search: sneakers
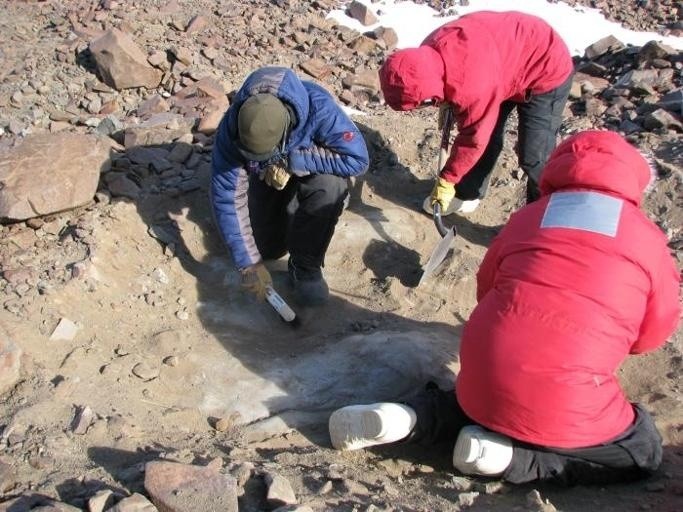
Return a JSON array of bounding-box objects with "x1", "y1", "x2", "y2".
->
[
  {"x1": 429, "y1": 176, "x2": 456, "y2": 213},
  {"x1": 423, "y1": 196, "x2": 481, "y2": 216},
  {"x1": 452, "y1": 425, "x2": 513, "y2": 477},
  {"x1": 329, "y1": 402, "x2": 417, "y2": 451},
  {"x1": 288, "y1": 256, "x2": 329, "y2": 305}
]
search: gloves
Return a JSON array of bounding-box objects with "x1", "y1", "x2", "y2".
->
[
  {"x1": 240, "y1": 263, "x2": 273, "y2": 304},
  {"x1": 260, "y1": 154, "x2": 294, "y2": 190}
]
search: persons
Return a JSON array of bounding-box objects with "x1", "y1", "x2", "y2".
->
[
  {"x1": 329, "y1": 132, "x2": 681, "y2": 487},
  {"x1": 378, "y1": 11, "x2": 573, "y2": 216},
  {"x1": 210, "y1": 67, "x2": 369, "y2": 307}
]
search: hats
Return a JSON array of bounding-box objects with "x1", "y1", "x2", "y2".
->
[{"x1": 231, "y1": 93, "x2": 291, "y2": 161}]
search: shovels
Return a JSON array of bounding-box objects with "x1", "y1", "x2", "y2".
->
[{"x1": 418, "y1": 108, "x2": 457, "y2": 288}]
